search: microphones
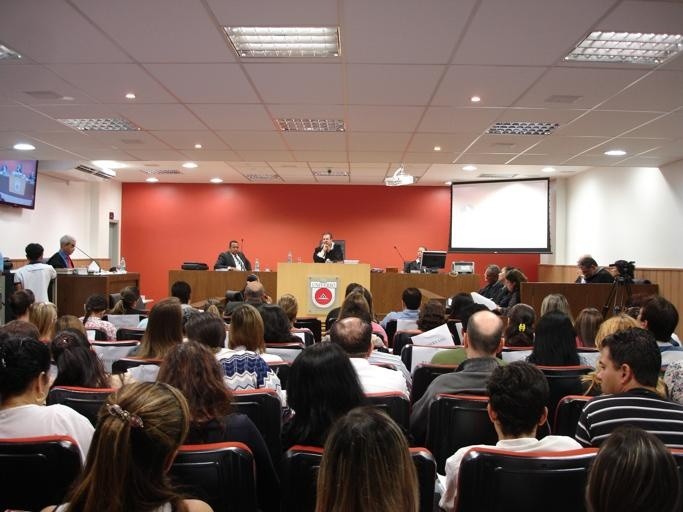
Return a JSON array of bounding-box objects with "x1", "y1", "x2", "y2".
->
[
  {"x1": 394, "y1": 246, "x2": 407, "y2": 273},
  {"x1": 241, "y1": 238, "x2": 244, "y2": 272},
  {"x1": 71, "y1": 244, "x2": 101, "y2": 275}
]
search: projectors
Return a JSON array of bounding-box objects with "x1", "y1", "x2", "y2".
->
[{"x1": 386, "y1": 175, "x2": 413, "y2": 187}]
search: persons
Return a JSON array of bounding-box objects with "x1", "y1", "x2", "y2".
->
[
  {"x1": 477, "y1": 265, "x2": 527, "y2": 313},
  {"x1": 1, "y1": 161, "x2": 35, "y2": 181},
  {"x1": 402, "y1": 246, "x2": 437, "y2": 273},
  {"x1": 313, "y1": 233, "x2": 343, "y2": 263},
  {"x1": 13, "y1": 243, "x2": 55, "y2": 302},
  {"x1": 214, "y1": 240, "x2": 252, "y2": 271},
  {"x1": 0, "y1": 274, "x2": 683, "y2": 512},
  {"x1": 47, "y1": 236, "x2": 76, "y2": 268},
  {"x1": 575, "y1": 255, "x2": 635, "y2": 283}
]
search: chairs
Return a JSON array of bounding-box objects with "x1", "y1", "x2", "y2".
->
[{"x1": 0, "y1": 308, "x2": 683, "y2": 512}]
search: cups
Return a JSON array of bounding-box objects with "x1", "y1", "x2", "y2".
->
[
  {"x1": 263, "y1": 265, "x2": 273, "y2": 272},
  {"x1": 297, "y1": 256, "x2": 301, "y2": 263},
  {"x1": 76, "y1": 268, "x2": 88, "y2": 275}
]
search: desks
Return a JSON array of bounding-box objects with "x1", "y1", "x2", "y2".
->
[
  {"x1": 168, "y1": 269, "x2": 277, "y2": 306},
  {"x1": 370, "y1": 271, "x2": 480, "y2": 317},
  {"x1": 58, "y1": 272, "x2": 140, "y2": 318},
  {"x1": 520, "y1": 282, "x2": 659, "y2": 320}
]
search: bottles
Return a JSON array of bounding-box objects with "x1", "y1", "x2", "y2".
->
[
  {"x1": 286, "y1": 248, "x2": 293, "y2": 262},
  {"x1": 119, "y1": 255, "x2": 125, "y2": 273},
  {"x1": 253, "y1": 255, "x2": 259, "y2": 272}
]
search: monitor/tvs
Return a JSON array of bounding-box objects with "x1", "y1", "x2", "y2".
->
[
  {"x1": 420, "y1": 251, "x2": 447, "y2": 273},
  {"x1": 0, "y1": 160, "x2": 38, "y2": 209}
]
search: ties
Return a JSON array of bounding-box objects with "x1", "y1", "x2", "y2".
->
[
  {"x1": 235, "y1": 255, "x2": 245, "y2": 271},
  {"x1": 66, "y1": 256, "x2": 73, "y2": 268}
]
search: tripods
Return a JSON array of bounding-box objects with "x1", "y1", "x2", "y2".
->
[{"x1": 602, "y1": 276, "x2": 632, "y2": 320}]
